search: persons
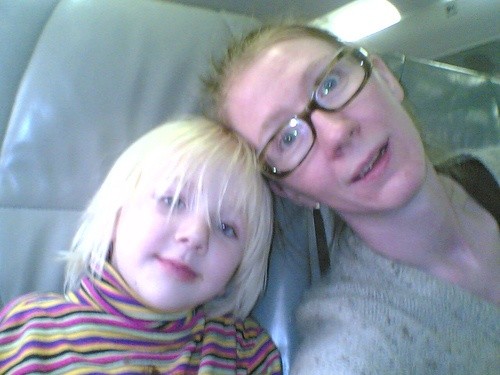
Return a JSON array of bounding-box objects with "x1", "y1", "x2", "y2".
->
[
  {"x1": 0, "y1": 115, "x2": 284, "y2": 375},
  {"x1": 196, "y1": 22, "x2": 500, "y2": 375}
]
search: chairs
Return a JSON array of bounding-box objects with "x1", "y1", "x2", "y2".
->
[{"x1": 1, "y1": 0, "x2": 500, "y2": 375}]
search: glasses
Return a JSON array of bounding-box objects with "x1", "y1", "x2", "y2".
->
[{"x1": 255, "y1": 44, "x2": 374, "y2": 183}]
